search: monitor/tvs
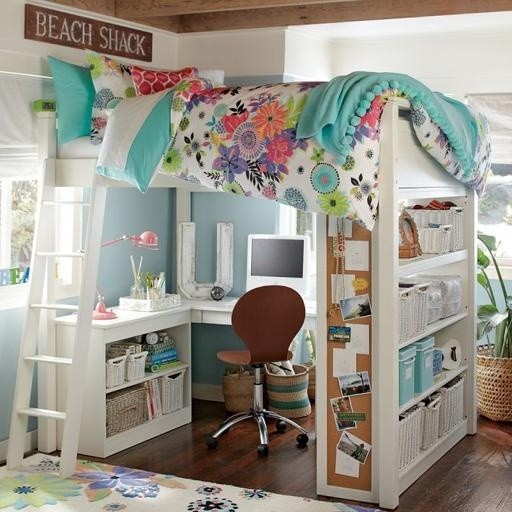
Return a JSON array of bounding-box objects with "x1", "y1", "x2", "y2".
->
[{"x1": 246, "y1": 233, "x2": 311, "y2": 299}]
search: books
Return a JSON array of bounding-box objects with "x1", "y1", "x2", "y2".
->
[
  {"x1": 107, "y1": 343, "x2": 141, "y2": 363},
  {"x1": 141, "y1": 379, "x2": 164, "y2": 421}
]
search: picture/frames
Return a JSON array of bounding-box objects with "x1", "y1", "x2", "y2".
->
[{"x1": 399, "y1": 211, "x2": 417, "y2": 245}]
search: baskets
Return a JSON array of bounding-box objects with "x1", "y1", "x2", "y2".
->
[
  {"x1": 105, "y1": 386, "x2": 145, "y2": 438},
  {"x1": 223, "y1": 370, "x2": 264, "y2": 412},
  {"x1": 422, "y1": 391, "x2": 444, "y2": 450},
  {"x1": 417, "y1": 222, "x2": 453, "y2": 255},
  {"x1": 105, "y1": 353, "x2": 127, "y2": 388},
  {"x1": 397, "y1": 404, "x2": 422, "y2": 474},
  {"x1": 405, "y1": 206, "x2": 464, "y2": 253},
  {"x1": 415, "y1": 281, "x2": 431, "y2": 335},
  {"x1": 124, "y1": 351, "x2": 149, "y2": 382},
  {"x1": 475, "y1": 341, "x2": 512, "y2": 424},
  {"x1": 160, "y1": 369, "x2": 186, "y2": 416},
  {"x1": 439, "y1": 374, "x2": 464, "y2": 438},
  {"x1": 399, "y1": 287, "x2": 416, "y2": 344},
  {"x1": 305, "y1": 363, "x2": 315, "y2": 399},
  {"x1": 264, "y1": 362, "x2": 315, "y2": 419}
]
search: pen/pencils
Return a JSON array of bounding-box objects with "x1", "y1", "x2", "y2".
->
[{"x1": 129, "y1": 254, "x2": 143, "y2": 300}]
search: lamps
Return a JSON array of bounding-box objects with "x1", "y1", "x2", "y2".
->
[{"x1": 80, "y1": 231, "x2": 159, "y2": 320}]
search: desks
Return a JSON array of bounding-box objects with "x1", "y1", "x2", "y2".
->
[{"x1": 192, "y1": 296, "x2": 316, "y2": 330}]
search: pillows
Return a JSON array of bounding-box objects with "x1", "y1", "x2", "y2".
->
[
  {"x1": 127, "y1": 65, "x2": 199, "y2": 97},
  {"x1": 46, "y1": 56, "x2": 96, "y2": 145},
  {"x1": 85, "y1": 48, "x2": 200, "y2": 147}
]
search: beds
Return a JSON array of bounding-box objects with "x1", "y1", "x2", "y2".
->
[{"x1": 34, "y1": 48, "x2": 493, "y2": 232}]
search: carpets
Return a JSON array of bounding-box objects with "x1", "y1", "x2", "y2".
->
[{"x1": 0, "y1": 453, "x2": 387, "y2": 512}]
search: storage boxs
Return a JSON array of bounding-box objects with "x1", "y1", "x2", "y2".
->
[
  {"x1": 399, "y1": 346, "x2": 416, "y2": 406},
  {"x1": 399, "y1": 246, "x2": 418, "y2": 258},
  {"x1": 409, "y1": 336, "x2": 434, "y2": 393}
]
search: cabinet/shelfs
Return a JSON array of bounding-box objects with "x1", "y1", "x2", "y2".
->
[
  {"x1": 54, "y1": 297, "x2": 192, "y2": 459},
  {"x1": 316, "y1": 188, "x2": 478, "y2": 508}
]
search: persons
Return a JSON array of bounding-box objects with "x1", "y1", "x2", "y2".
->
[
  {"x1": 348, "y1": 439, "x2": 365, "y2": 459},
  {"x1": 337, "y1": 398, "x2": 355, "y2": 429}
]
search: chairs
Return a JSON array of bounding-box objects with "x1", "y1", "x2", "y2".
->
[{"x1": 204, "y1": 285, "x2": 309, "y2": 458}]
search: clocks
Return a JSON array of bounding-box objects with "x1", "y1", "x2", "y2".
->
[{"x1": 209, "y1": 286, "x2": 225, "y2": 304}]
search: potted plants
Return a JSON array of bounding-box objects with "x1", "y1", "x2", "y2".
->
[{"x1": 477, "y1": 230, "x2": 512, "y2": 423}]
